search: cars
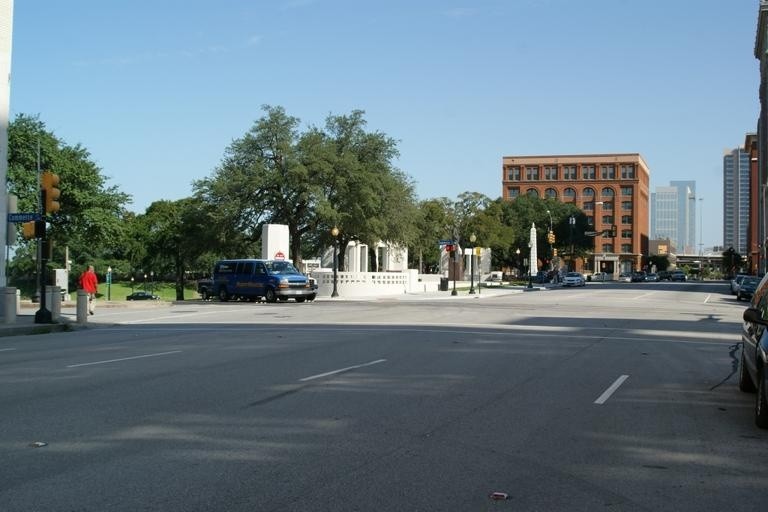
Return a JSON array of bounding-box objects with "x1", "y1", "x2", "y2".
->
[
  {"x1": 618, "y1": 270, "x2": 686, "y2": 283},
  {"x1": 197, "y1": 274, "x2": 246, "y2": 300},
  {"x1": 730, "y1": 272, "x2": 768, "y2": 427},
  {"x1": 280, "y1": 277, "x2": 318, "y2": 301},
  {"x1": 537, "y1": 272, "x2": 609, "y2": 286},
  {"x1": 127, "y1": 292, "x2": 159, "y2": 300}
]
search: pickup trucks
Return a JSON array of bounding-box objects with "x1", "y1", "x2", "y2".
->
[{"x1": 483, "y1": 271, "x2": 514, "y2": 282}]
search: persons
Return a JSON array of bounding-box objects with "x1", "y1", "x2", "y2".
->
[{"x1": 79, "y1": 266, "x2": 98, "y2": 315}]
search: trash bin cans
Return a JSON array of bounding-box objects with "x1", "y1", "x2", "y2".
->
[
  {"x1": 587, "y1": 275, "x2": 591, "y2": 281},
  {"x1": 441, "y1": 278, "x2": 448, "y2": 291}
]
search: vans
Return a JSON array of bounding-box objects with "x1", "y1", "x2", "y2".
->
[{"x1": 215, "y1": 258, "x2": 319, "y2": 303}]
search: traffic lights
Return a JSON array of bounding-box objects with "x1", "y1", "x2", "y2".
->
[
  {"x1": 42, "y1": 239, "x2": 54, "y2": 261},
  {"x1": 552, "y1": 248, "x2": 558, "y2": 257},
  {"x1": 20, "y1": 222, "x2": 45, "y2": 240},
  {"x1": 46, "y1": 173, "x2": 62, "y2": 213},
  {"x1": 547, "y1": 232, "x2": 556, "y2": 244}
]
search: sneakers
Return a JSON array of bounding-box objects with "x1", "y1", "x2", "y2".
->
[{"x1": 86, "y1": 310, "x2": 94, "y2": 316}]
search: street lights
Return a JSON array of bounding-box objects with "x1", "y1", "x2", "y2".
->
[
  {"x1": 546, "y1": 209, "x2": 554, "y2": 270},
  {"x1": 332, "y1": 225, "x2": 340, "y2": 298},
  {"x1": 527, "y1": 240, "x2": 533, "y2": 286},
  {"x1": 595, "y1": 201, "x2": 608, "y2": 253},
  {"x1": 105, "y1": 266, "x2": 112, "y2": 300},
  {"x1": 131, "y1": 271, "x2": 154, "y2": 293},
  {"x1": 516, "y1": 248, "x2": 521, "y2": 283},
  {"x1": 469, "y1": 232, "x2": 477, "y2": 294}
]
